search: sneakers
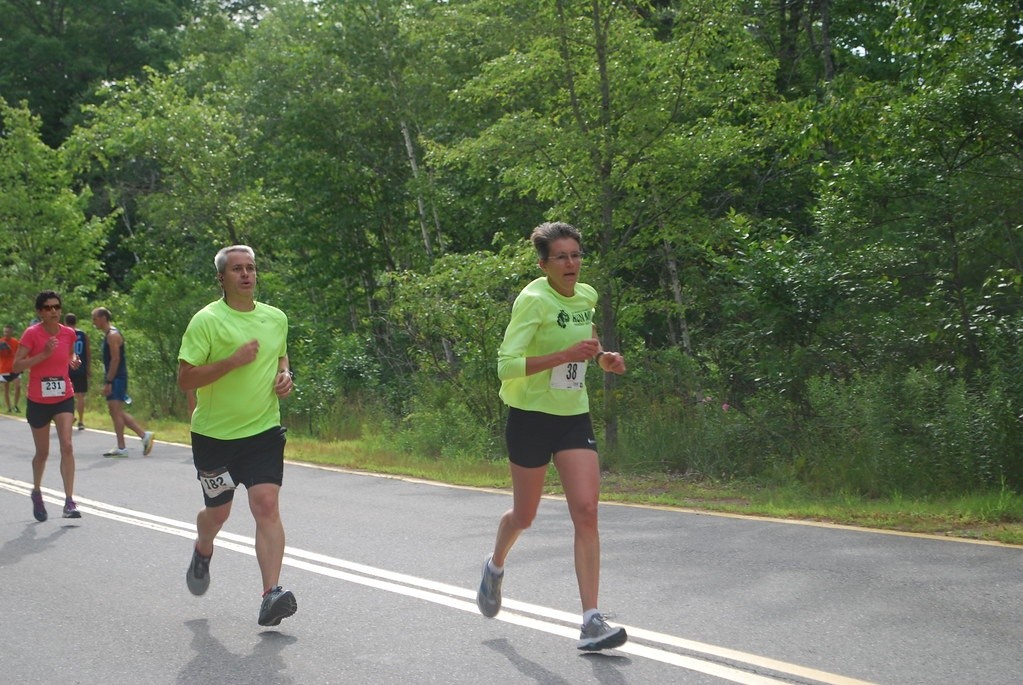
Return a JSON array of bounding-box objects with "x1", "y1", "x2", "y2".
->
[
  {"x1": 103, "y1": 447, "x2": 129, "y2": 458},
  {"x1": 31, "y1": 489, "x2": 48, "y2": 522},
  {"x1": 476, "y1": 553, "x2": 504, "y2": 617},
  {"x1": 186, "y1": 536, "x2": 214, "y2": 595},
  {"x1": 257, "y1": 585, "x2": 297, "y2": 626},
  {"x1": 578, "y1": 612, "x2": 627, "y2": 651},
  {"x1": 62, "y1": 500, "x2": 82, "y2": 518},
  {"x1": 142, "y1": 432, "x2": 155, "y2": 455}
]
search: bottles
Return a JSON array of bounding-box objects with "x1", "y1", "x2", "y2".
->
[{"x1": 125, "y1": 395, "x2": 132, "y2": 404}]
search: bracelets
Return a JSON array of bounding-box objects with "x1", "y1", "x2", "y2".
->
[
  {"x1": 280, "y1": 369, "x2": 293, "y2": 380},
  {"x1": 104, "y1": 379, "x2": 113, "y2": 384},
  {"x1": 595, "y1": 352, "x2": 604, "y2": 366}
]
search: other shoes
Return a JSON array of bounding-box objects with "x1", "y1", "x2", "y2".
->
[
  {"x1": 7, "y1": 409, "x2": 11, "y2": 413},
  {"x1": 78, "y1": 422, "x2": 84, "y2": 430},
  {"x1": 14, "y1": 405, "x2": 21, "y2": 413}
]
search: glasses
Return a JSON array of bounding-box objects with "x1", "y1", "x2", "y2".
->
[
  {"x1": 547, "y1": 251, "x2": 582, "y2": 263},
  {"x1": 44, "y1": 304, "x2": 60, "y2": 310}
]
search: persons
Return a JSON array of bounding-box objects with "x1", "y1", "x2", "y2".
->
[
  {"x1": 477, "y1": 221, "x2": 628, "y2": 652},
  {"x1": 12, "y1": 291, "x2": 81, "y2": 522},
  {"x1": 0, "y1": 313, "x2": 90, "y2": 430},
  {"x1": 177, "y1": 246, "x2": 296, "y2": 627},
  {"x1": 91, "y1": 307, "x2": 155, "y2": 458}
]
